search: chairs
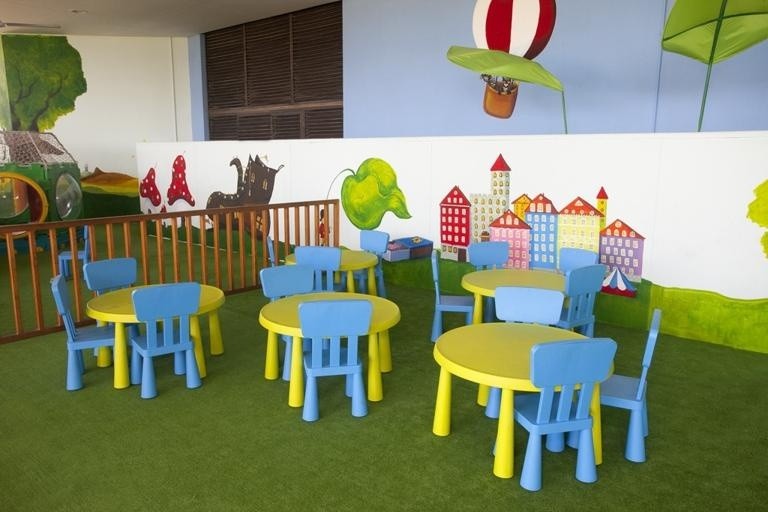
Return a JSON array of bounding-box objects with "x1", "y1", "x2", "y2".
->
[{"x1": 49, "y1": 223, "x2": 204, "y2": 399}]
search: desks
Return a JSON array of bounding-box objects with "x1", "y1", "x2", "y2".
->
[{"x1": 83, "y1": 282, "x2": 228, "y2": 391}]
